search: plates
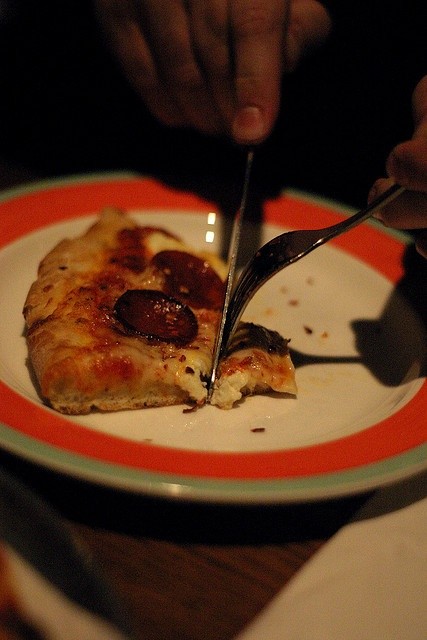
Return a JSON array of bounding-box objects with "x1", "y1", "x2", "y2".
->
[{"x1": 1, "y1": 173, "x2": 427, "y2": 503}]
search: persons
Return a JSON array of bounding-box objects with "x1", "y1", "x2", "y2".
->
[{"x1": 105, "y1": 0, "x2": 427, "y2": 256}]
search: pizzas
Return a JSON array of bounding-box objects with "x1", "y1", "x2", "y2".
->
[{"x1": 22, "y1": 205, "x2": 300, "y2": 414}]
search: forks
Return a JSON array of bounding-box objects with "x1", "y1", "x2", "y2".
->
[{"x1": 225, "y1": 186, "x2": 404, "y2": 353}]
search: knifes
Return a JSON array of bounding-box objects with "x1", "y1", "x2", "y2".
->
[{"x1": 208, "y1": 153, "x2": 253, "y2": 400}]
